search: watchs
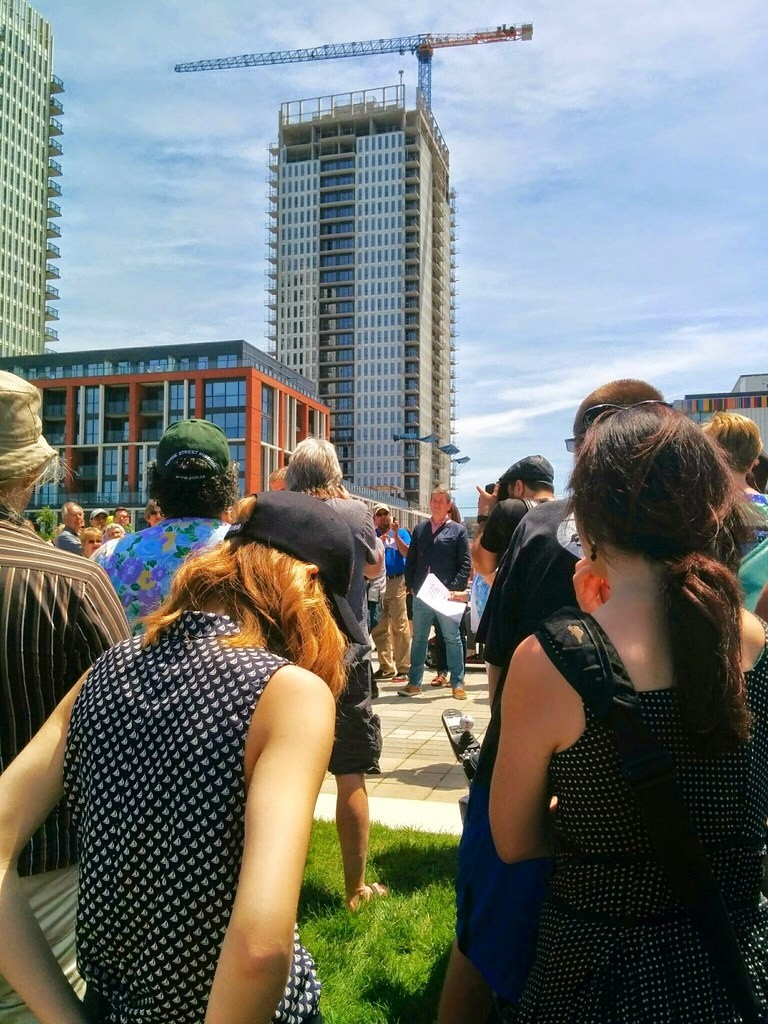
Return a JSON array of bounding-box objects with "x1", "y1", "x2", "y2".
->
[{"x1": 477, "y1": 515, "x2": 490, "y2": 524}]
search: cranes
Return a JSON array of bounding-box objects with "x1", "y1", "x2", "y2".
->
[{"x1": 175, "y1": 21, "x2": 533, "y2": 120}]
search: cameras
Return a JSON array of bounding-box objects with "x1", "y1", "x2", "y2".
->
[{"x1": 485, "y1": 483, "x2": 509, "y2": 503}]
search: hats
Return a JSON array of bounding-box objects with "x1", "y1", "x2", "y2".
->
[
  {"x1": 90, "y1": 509, "x2": 109, "y2": 519},
  {"x1": 373, "y1": 503, "x2": 389, "y2": 515},
  {"x1": 0, "y1": 369, "x2": 59, "y2": 484},
  {"x1": 499, "y1": 455, "x2": 554, "y2": 483},
  {"x1": 156, "y1": 419, "x2": 230, "y2": 480},
  {"x1": 223, "y1": 490, "x2": 368, "y2": 645}
]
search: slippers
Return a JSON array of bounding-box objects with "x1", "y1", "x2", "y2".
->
[{"x1": 366, "y1": 883, "x2": 390, "y2": 897}]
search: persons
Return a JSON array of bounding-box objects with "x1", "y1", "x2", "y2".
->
[
  {"x1": 434, "y1": 380, "x2": 667, "y2": 1024},
  {"x1": 23, "y1": 410, "x2": 768, "y2": 700},
  {"x1": 0, "y1": 491, "x2": 368, "y2": 1023},
  {"x1": 488, "y1": 402, "x2": 767, "y2": 1023},
  {"x1": 0, "y1": 374, "x2": 137, "y2": 1022},
  {"x1": 284, "y1": 438, "x2": 391, "y2": 913}
]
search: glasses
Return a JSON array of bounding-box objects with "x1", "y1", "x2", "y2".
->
[
  {"x1": 564, "y1": 437, "x2": 575, "y2": 452},
  {"x1": 88, "y1": 539, "x2": 102, "y2": 543},
  {"x1": 582, "y1": 398, "x2": 673, "y2": 427},
  {"x1": 376, "y1": 511, "x2": 389, "y2": 516},
  {"x1": 150, "y1": 510, "x2": 163, "y2": 515}
]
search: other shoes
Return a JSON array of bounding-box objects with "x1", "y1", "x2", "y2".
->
[
  {"x1": 397, "y1": 685, "x2": 421, "y2": 696},
  {"x1": 393, "y1": 673, "x2": 407, "y2": 680},
  {"x1": 453, "y1": 687, "x2": 467, "y2": 700},
  {"x1": 373, "y1": 670, "x2": 393, "y2": 680},
  {"x1": 431, "y1": 674, "x2": 446, "y2": 686}
]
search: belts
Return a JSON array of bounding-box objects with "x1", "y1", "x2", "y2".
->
[{"x1": 387, "y1": 572, "x2": 403, "y2": 580}]
